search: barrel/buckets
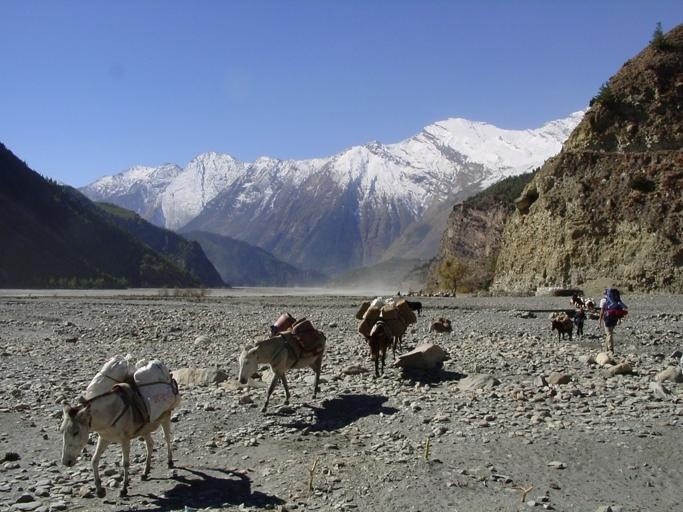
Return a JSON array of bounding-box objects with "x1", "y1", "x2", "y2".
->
[
  {"x1": 270, "y1": 312, "x2": 293, "y2": 330},
  {"x1": 290, "y1": 318, "x2": 322, "y2": 352}
]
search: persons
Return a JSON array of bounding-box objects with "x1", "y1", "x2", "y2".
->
[
  {"x1": 596, "y1": 287, "x2": 621, "y2": 355},
  {"x1": 573, "y1": 306, "x2": 586, "y2": 337}
]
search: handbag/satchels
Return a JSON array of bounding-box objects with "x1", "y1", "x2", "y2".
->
[{"x1": 605, "y1": 309, "x2": 628, "y2": 318}]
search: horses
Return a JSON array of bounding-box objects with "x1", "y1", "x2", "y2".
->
[
  {"x1": 60, "y1": 380, "x2": 179, "y2": 499},
  {"x1": 359, "y1": 299, "x2": 424, "y2": 380},
  {"x1": 238, "y1": 329, "x2": 327, "y2": 413},
  {"x1": 552, "y1": 318, "x2": 574, "y2": 342}
]
